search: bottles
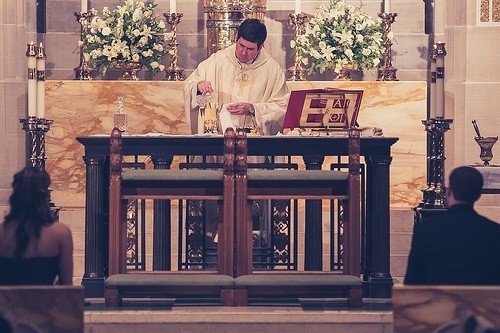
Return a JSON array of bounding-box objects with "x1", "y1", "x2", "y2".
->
[{"x1": 113, "y1": 96, "x2": 127, "y2": 135}]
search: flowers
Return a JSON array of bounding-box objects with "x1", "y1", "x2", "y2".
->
[
  {"x1": 71, "y1": 0, "x2": 175, "y2": 72},
  {"x1": 289, "y1": 0, "x2": 389, "y2": 76}
]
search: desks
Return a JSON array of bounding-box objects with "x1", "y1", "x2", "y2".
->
[{"x1": 74, "y1": 135, "x2": 399, "y2": 311}]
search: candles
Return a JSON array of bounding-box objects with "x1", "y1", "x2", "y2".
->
[
  {"x1": 430, "y1": 42, "x2": 446, "y2": 118},
  {"x1": 295, "y1": 0, "x2": 302, "y2": 14},
  {"x1": 384, "y1": 0, "x2": 391, "y2": 13},
  {"x1": 81, "y1": 0, "x2": 88, "y2": 13},
  {"x1": 25, "y1": 40, "x2": 45, "y2": 119},
  {"x1": 170, "y1": 0, "x2": 177, "y2": 16}
]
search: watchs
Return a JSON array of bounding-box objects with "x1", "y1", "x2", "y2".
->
[{"x1": 249, "y1": 107, "x2": 255, "y2": 113}]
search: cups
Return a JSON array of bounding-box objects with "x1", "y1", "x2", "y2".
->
[{"x1": 199, "y1": 103, "x2": 223, "y2": 134}]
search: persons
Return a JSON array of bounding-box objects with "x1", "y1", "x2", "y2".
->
[
  {"x1": 183, "y1": 19, "x2": 291, "y2": 171},
  {"x1": 403, "y1": 166, "x2": 500, "y2": 286},
  {"x1": 0, "y1": 163, "x2": 74, "y2": 287}
]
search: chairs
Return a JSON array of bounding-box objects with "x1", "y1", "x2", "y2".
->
[
  {"x1": 0, "y1": 285, "x2": 85, "y2": 333},
  {"x1": 390, "y1": 285, "x2": 500, "y2": 333}
]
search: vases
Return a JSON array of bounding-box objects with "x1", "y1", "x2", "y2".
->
[
  {"x1": 114, "y1": 60, "x2": 143, "y2": 80},
  {"x1": 328, "y1": 61, "x2": 356, "y2": 81}
]
